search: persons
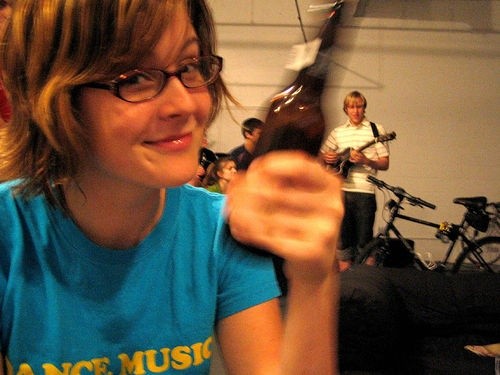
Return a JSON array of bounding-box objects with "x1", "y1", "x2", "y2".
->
[
  {"x1": 187, "y1": 118, "x2": 268, "y2": 200},
  {"x1": 0, "y1": 0, "x2": 344, "y2": 375},
  {"x1": 320, "y1": 90, "x2": 389, "y2": 271}
]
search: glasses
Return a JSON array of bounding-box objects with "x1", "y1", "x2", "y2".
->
[{"x1": 75, "y1": 54, "x2": 223, "y2": 103}]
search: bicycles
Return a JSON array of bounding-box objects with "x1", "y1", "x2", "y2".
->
[
  {"x1": 356, "y1": 175, "x2": 500, "y2": 271},
  {"x1": 461, "y1": 202, "x2": 500, "y2": 266}
]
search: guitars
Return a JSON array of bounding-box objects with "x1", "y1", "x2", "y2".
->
[{"x1": 328, "y1": 131, "x2": 396, "y2": 179}]
